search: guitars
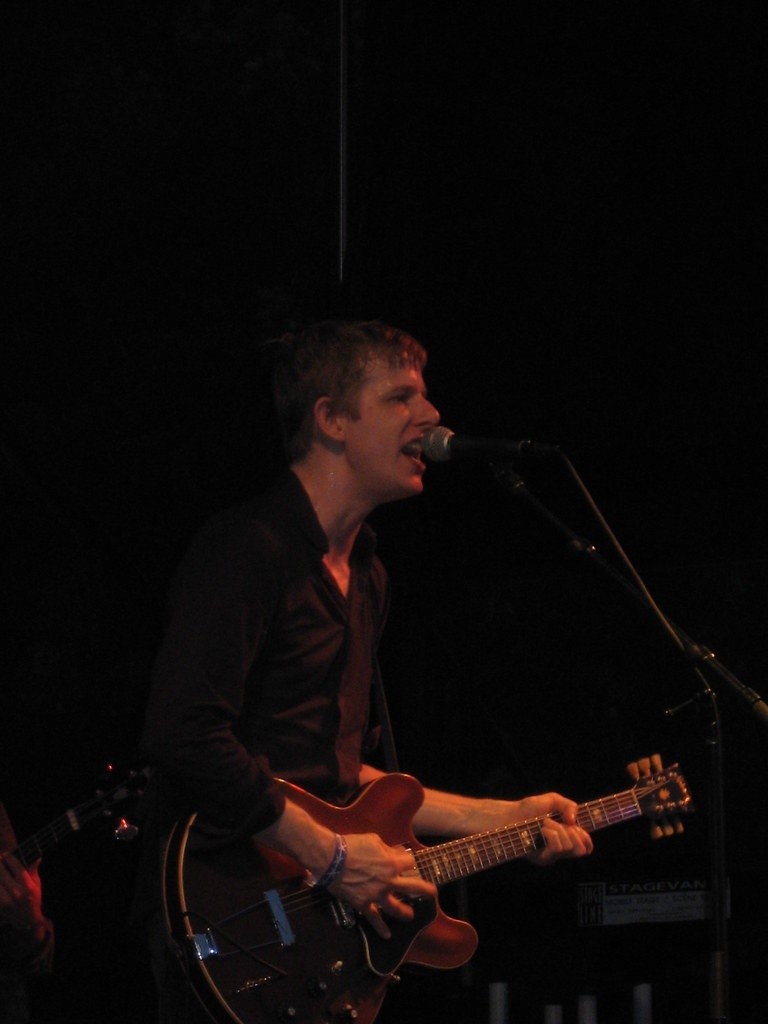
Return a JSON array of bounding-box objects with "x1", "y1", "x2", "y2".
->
[
  {"x1": 161, "y1": 750, "x2": 696, "y2": 1024},
  {"x1": 8, "y1": 767, "x2": 152, "y2": 891}
]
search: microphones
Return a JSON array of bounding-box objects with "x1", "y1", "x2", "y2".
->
[{"x1": 422, "y1": 426, "x2": 559, "y2": 462}]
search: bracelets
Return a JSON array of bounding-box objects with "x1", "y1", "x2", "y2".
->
[
  {"x1": 27, "y1": 919, "x2": 56, "y2": 966},
  {"x1": 311, "y1": 834, "x2": 347, "y2": 889}
]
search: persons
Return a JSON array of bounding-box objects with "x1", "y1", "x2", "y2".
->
[
  {"x1": 0, "y1": 801, "x2": 48, "y2": 1024},
  {"x1": 138, "y1": 320, "x2": 594, "y2": 1023}
]
